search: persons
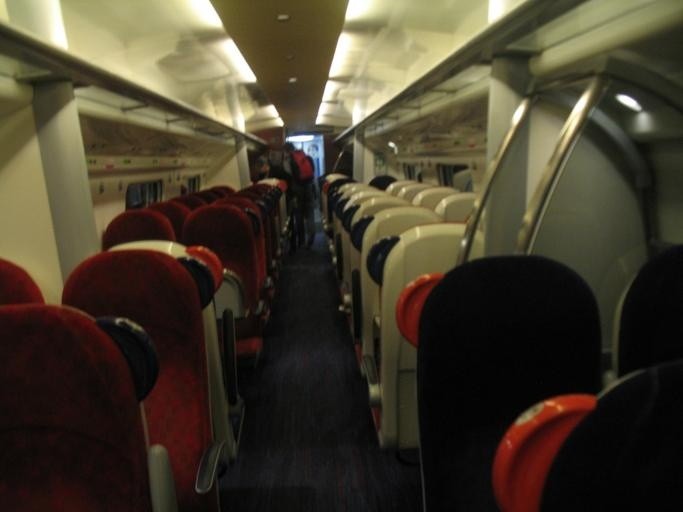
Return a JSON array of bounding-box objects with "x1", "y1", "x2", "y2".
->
[{"x1": 250, "y1": 135, "x2": 322, "y2": 255}]
